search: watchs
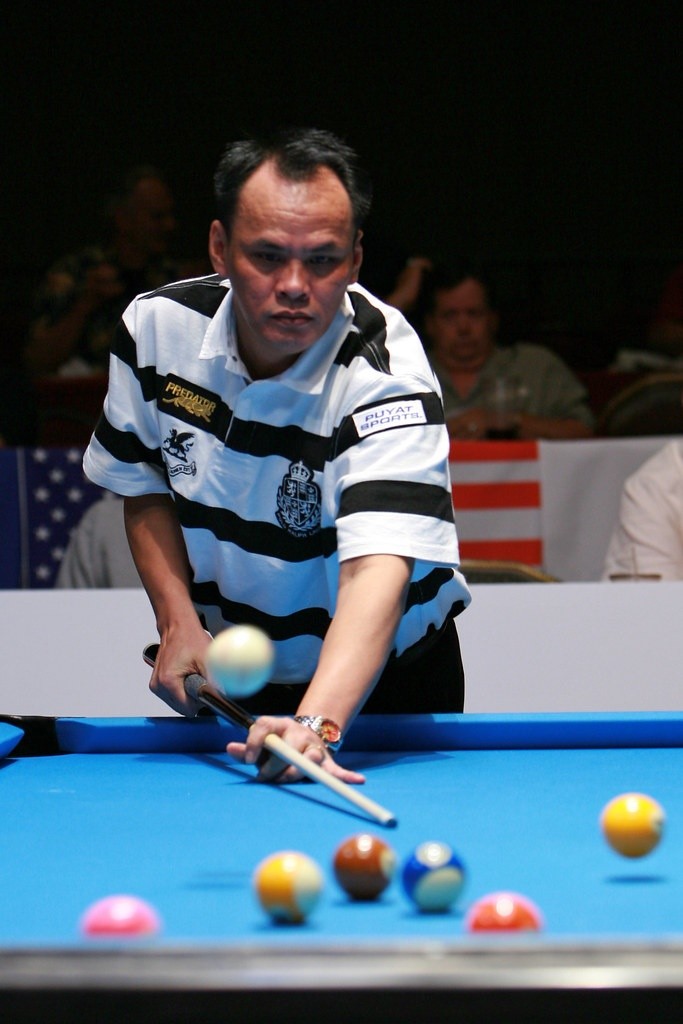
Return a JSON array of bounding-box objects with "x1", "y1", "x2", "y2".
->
[{"x1": 292, "y1": 715, "x2": 342, "y2": 753}]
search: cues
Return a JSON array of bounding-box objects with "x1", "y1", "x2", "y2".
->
[{"x1": 144, "y1": 642, "x2": 396, "y2": 824}]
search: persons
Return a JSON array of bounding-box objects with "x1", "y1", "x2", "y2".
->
[
  {"x1": 21, "y1": 164, "x2": 683, "y2": 589},
  {"x1": 83, "y1": 131, "x2": 473, "y2": 786}
]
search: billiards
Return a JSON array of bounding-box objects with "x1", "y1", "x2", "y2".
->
[
  {"x1": 81, "y1": 894, "x2": 156, "y2": 940},
  {"x1": 206, "y1": 622, "x2": 271, "y2": 697},
  {"x1": 333, "y1": 833, "x2": 393, "y2": 902},
  {"x1": 601, "y1": 789, "x2": 666, "y2": 860},
  {"x1": 468, "y1": 890, "x2": 542, "y2": 933},
  {"x1": 254, "y1": 851, "x2": 323, "y2": 922},
  {"x1": 402, "y1": 841, "x2": 466, "y2": 912}
]
